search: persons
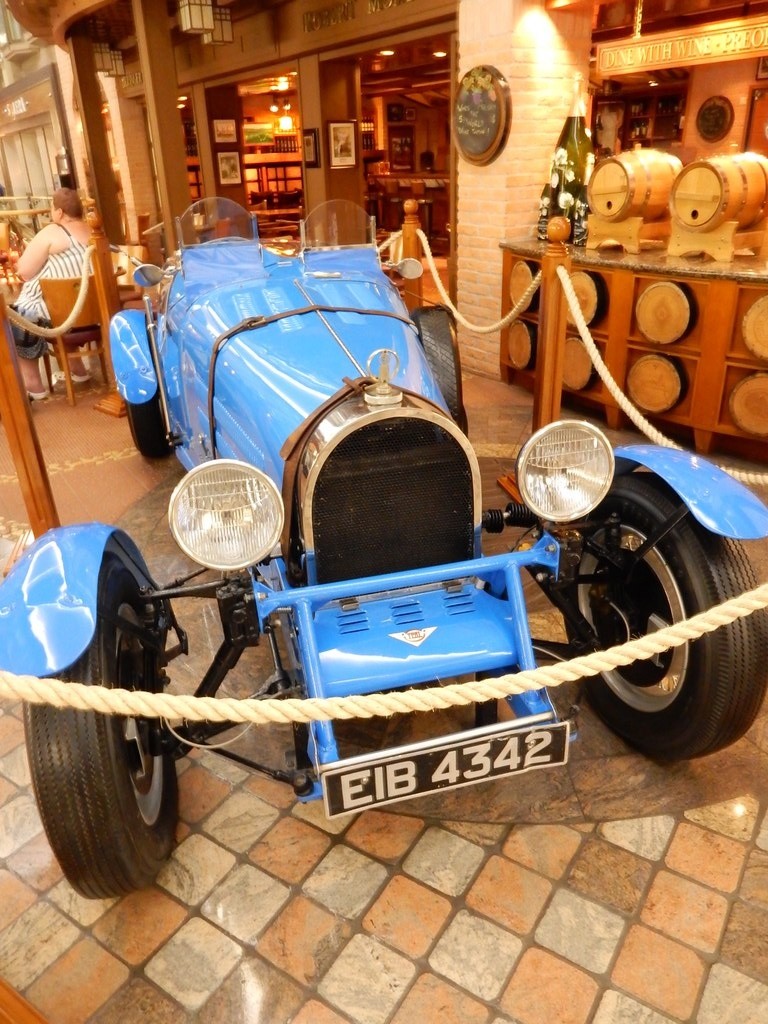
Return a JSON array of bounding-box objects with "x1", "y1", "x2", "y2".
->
[{"x1": 8, "y1": 187, "x2": 94, "y2": 402}]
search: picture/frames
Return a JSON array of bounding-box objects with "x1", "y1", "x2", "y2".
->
[
  {"x1": 303, "y1": 128, "x2": 320, "y2": 167},
  {"x1": 212, "y1": 118, "x2": 238, "y2": 144},
  {"x1": 216, "y1": 151, "x2": 243, "y2": 186},
  {"x1": 325, "y1": 120, "x2": 359, "y2": 169},
  {"x1": 756, "y1": 56, "x2": 768, "y2": 82}
]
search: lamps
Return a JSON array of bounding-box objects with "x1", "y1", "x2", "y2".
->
[
  {"x1": 93, "y1": 43, "x2": 127, "y2": 78},
  {"x1": 178, "y1": 0, "x2": 234, "y2": 45}
]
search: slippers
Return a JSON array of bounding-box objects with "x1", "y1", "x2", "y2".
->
[
  {"x1": 26, "y1": 391, "x2": 49, "y2": 399},
  {"x1": 54, "y1": 371, "x2": 90, "y2": 382}
]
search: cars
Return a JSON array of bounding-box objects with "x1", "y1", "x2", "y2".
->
[{"x1": 0, "y1": 196, "x2": 768, "y2": 899}]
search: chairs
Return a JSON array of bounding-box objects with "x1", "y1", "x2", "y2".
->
[
  {"x1": 0, "y1": 222, "x2": 19, "y2": 287},
  {"x1": 250, "y1": 188, "x2": 302, "y2": 244},
  {"x1": 110, "y1": 244, "x2": 150, "y2": 302},
  {"x1": 34, "y1": 275, "x2": 109, "y2": 406}
]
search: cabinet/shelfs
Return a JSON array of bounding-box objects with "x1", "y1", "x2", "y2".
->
[
  {"x1": 386, "y1": 124, "x2": 415, "y2": 170},
  {"x1": 589, "y1": 84, "x2": 688, "y2": 158}
]
